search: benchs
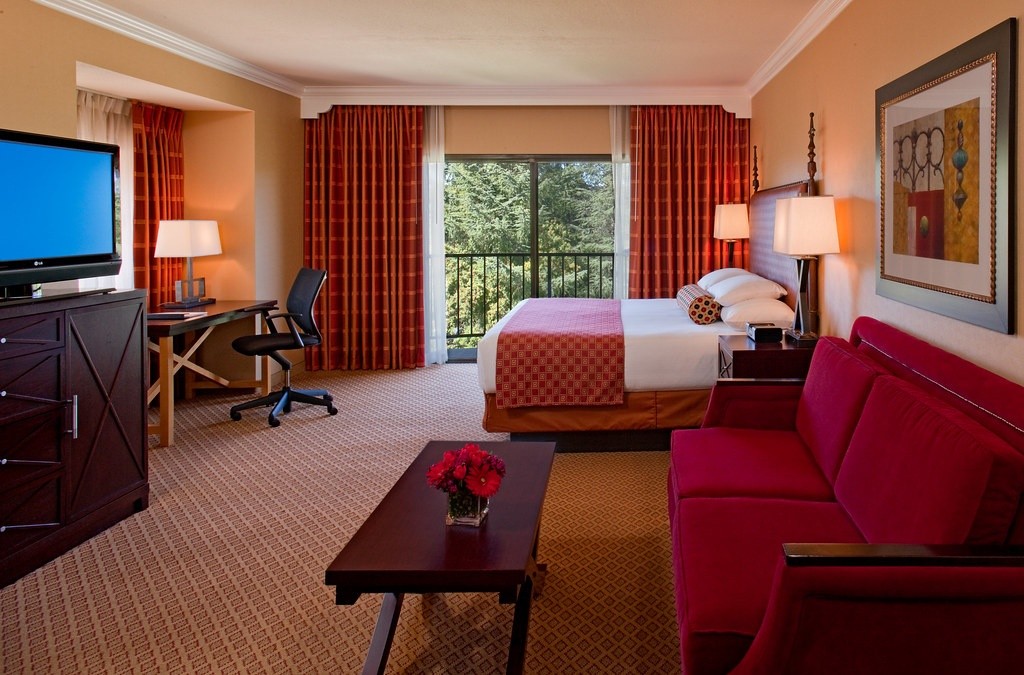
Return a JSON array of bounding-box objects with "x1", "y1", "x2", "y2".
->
[{"x1": 670, "y1": 315, "x2": 1024, "y2": 675}]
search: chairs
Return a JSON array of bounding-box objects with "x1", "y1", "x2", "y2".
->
[{"x1": 230, "y1": 267, "x2": 339, "y2": 427}]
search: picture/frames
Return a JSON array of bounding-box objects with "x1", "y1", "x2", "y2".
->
[{"x1": 875, "y1": 17, "x2": 1016, "y2": 335}]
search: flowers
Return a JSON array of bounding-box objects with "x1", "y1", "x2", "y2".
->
[{"x1": 426, "y1": 442, "x2": 508, "y2": 496}]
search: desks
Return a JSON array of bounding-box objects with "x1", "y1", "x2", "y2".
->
[
  {"x1": 325, "y1": 440, "x2": 555, "y2": 675},
  {"x1": 717, "y1": 335, "x2": 817, "y2": 380},
  {"x1": 147, "y1": 299, "x2": 278, "y2": 447}
]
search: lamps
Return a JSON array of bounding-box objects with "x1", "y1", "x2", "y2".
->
[
  {"x1": 714, "y1": 204, "x2": 751, "y2": 268},
  {"x1": 153, "y1": 220, "x2": 222, "y2": 308},
  {"x1": 773, "y1": 196, "x2": 840, "y2": 346}
]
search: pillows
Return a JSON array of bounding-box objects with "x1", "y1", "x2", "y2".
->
[{"x1": 676, "y1": 267, "x2": 795, "y2": 331}]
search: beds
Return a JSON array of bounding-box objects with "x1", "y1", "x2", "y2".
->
[{"x1": 476, "y1": 182, "x2": 816, "y2": 442}]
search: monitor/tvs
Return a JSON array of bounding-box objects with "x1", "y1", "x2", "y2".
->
[{"x1": 0, "y1": 128, "x2": 123, "y2": 309}]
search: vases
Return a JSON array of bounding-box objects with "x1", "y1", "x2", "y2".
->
[{"x1": 446, "y1": 487, "x2": 489, "y2": 525}]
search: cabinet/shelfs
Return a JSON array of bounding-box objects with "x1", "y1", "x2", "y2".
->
[{"x1": 0, "y1": 288, "x2": 148, "y2": 592}]
